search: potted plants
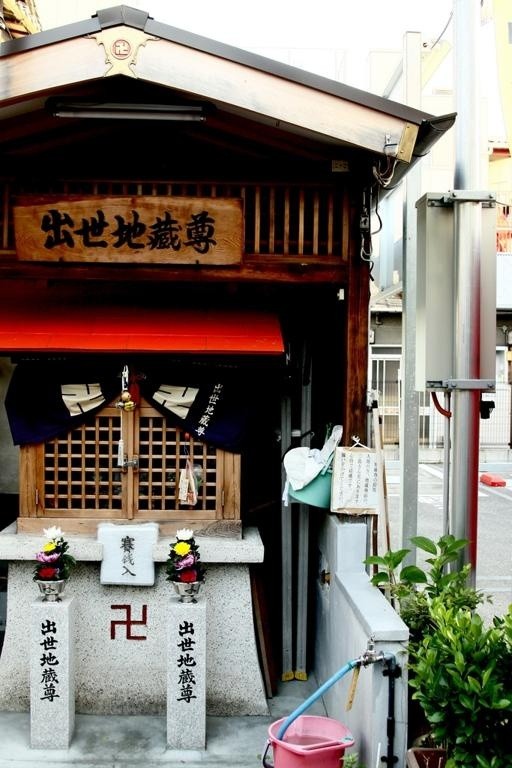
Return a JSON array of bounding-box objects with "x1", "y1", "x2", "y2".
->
[{"x1": 399, "y1": 591, "x2": 511, "y2": 768}]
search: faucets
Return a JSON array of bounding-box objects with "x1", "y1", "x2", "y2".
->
[{"x1": 360, "y1": 634, "x2": 382, "y2": 666}]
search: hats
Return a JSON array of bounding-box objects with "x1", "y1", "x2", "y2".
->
[{"x1": 283, "y1": 447, "x2": 325, "y2": 491}]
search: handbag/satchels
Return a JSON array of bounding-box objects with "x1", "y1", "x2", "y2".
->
[{"x1": 330, "y1": 436, "x2": 384, "y2": 516}]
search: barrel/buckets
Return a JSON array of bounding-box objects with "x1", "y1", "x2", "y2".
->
[
  {"x1": 262, "y1": 713, "x2": 357, "y2": 767},
  {"x1": 287, "y1": 443, "x2": 337, "y2": 507}
]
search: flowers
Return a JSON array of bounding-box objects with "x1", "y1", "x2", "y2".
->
[
  {"x1": 166, "y1": 527, "x2": 208, "y2": 584},
  {"x1": 31, "y1": 524, "x2": 76, "y2": 580}
]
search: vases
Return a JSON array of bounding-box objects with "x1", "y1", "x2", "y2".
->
[
  {"x1": 172, "y1": 579, "x2": 206, "y2": 603},
  {"x1": 35, "y1": 577, "x2": 68, "y2": 602}
]
